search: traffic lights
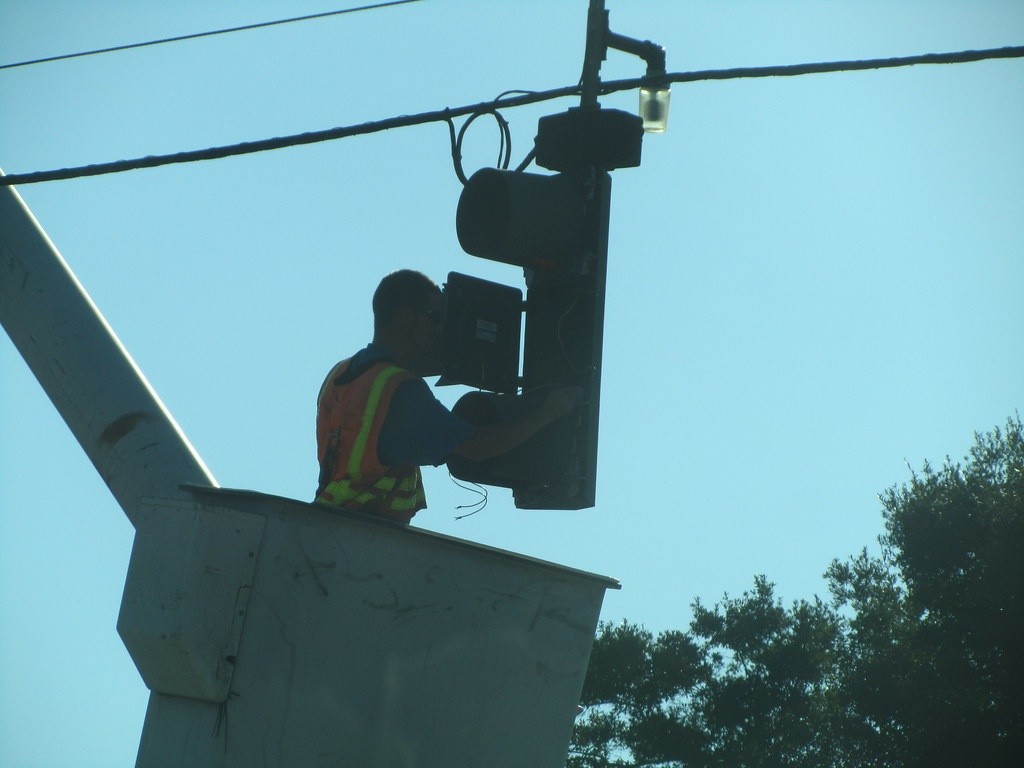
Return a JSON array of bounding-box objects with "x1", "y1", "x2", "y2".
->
[{"x1": 446, "y1": 169, "x2": 613, "y2": 510}]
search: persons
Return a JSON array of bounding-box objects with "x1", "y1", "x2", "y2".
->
[{"x1": 314, "y1": 269, "x2": 584, "y2": 525}]
